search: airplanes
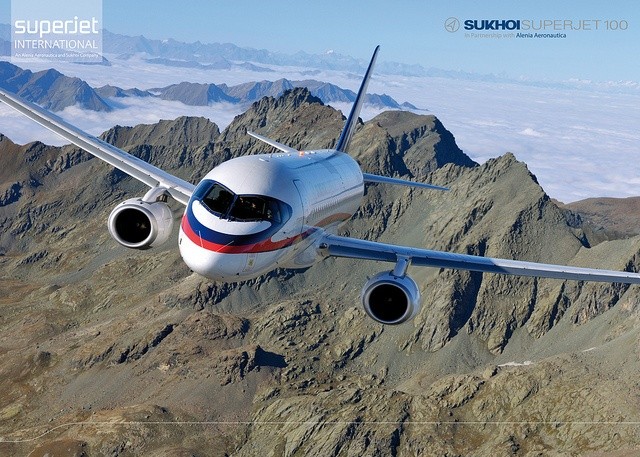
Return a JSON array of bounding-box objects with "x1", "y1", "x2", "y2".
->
[{"x1": 0, "y1": 45, "x2": 640, "y2": 325}]
